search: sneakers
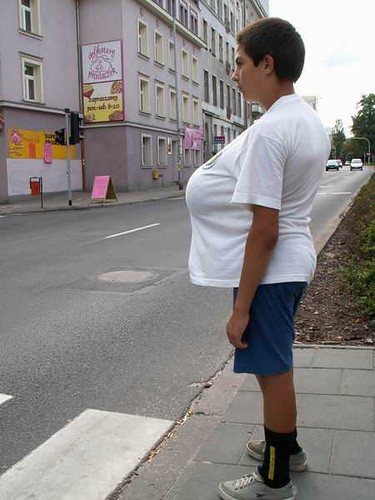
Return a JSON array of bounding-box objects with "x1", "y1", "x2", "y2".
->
[
  {"x1": 218, "y1": 466, "x2": 298, "y2": 500},
  {"x1": 246, "y1": 439, "x2": 308, "y2": 472}
]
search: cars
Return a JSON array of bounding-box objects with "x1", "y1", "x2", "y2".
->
[
  {"x1": 350, "y1": 159, "x2": 362, "y2": 171},
  {"x1": 326, "y1": 160, "x2": 342, "y2": 171},
  {"x1": 344, "y1": 161, "x2": 349, "y2": 166}
]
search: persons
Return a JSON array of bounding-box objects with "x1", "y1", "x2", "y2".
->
[{"x1": 185, "y1": 17, "x2": 330, "y2": 499}]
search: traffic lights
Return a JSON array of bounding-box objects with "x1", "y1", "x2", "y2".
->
[
  {"x1": 69, "y1": 112, "x2": 84, "y2": 145},
  {"x1": 55, "y1": 129, "x2": 66, "y2": 145}
]
